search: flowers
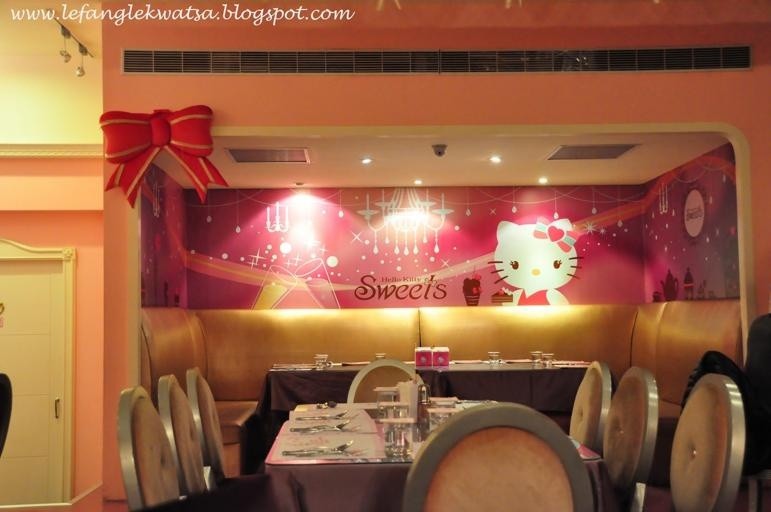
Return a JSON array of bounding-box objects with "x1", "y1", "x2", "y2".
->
[{"x1": 463, "y1": 265, "x2": 482, "y2": 295}]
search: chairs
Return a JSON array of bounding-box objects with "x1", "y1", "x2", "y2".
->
[
  {"x1": 402, "y1": 404, "x2": 595, "y2": 512},
  {"x1": 0, "y1": 373, "x2": 12, "y2": 457},
  {"x1": 570, "y1": 360, "x2": 612, "y2": 458},
  {"x1": 158, "y1": 374, "x2": 270, "y2": 499},
  {"x1": 603, "y1": 366, "x2": 659, "y2": 512},
  {"x1": 116, "y1": 386, "x2": 270, "y2": 511},
  {"x1": 185, "y1": 368, "x2": 270, "y2": 488},
  {"x1": 669, "y1": 373, "x2": 746, "y2": 512}
]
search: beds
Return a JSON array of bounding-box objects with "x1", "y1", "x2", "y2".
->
[{"x1": 245, "y1": 361, "x2": 619, "y2": 458}]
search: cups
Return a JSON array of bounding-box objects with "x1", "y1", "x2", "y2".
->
[
  {"x1": 312, "y1": 353, "x2": 328, "y2": 370},
  {"x1": 488, "y1": 351, "x2": 502, "y2": 366},
  {"x1": 530, "y1": 352, "x2": 554, "y2": 366},
  {"x1": 378, "y1": 388, "x2": 414, "y2": 461}
]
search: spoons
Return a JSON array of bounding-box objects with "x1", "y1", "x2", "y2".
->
[{"x1": 282, "y1": 410, "x2": 355, "y2": 457}]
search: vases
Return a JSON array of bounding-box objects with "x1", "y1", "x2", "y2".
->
[{"x1": 463, "y1": 293, "x2": 480, "y2": 305}]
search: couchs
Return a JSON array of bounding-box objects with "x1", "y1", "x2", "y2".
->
[{"x1": 140, "y1": 300, "x2": 741, "y2": 478}]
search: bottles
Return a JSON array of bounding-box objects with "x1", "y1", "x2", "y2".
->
[
  {"x1": 374, "y1": 352, "x2": 386, "y2": 361},
  {"x1": 418, "y1": 384, "x2": 431, "y2": 403}
]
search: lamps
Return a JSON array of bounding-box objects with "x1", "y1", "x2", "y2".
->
[{"x1": 47, "y1": 8, "x2": 94, "y2": 76}]
